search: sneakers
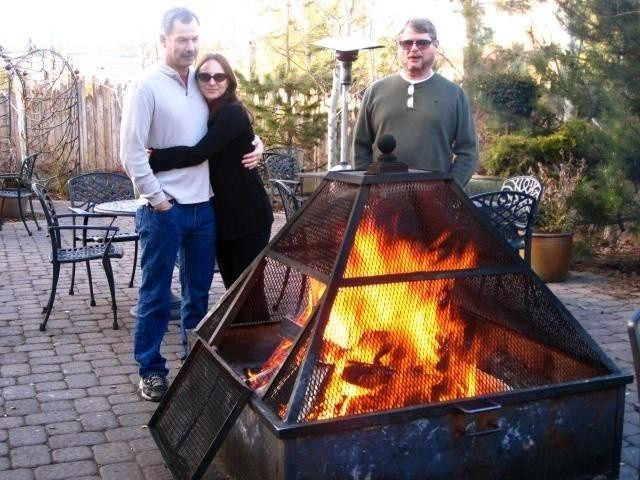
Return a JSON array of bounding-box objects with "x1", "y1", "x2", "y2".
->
[{"x1": 139, "y1": 375, "x2": 167, "y2": 401}]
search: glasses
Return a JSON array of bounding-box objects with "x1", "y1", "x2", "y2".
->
[
  {"x1": 197, "y1": 72, "x2": 228, "y2": 82},
  {"x1": 397, "y1": 40, "x2": 432, "y2": 50}
]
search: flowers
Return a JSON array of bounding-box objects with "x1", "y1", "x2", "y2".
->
[{"x1": 523, "y1": 154, "x2": 588, "y2": 233}]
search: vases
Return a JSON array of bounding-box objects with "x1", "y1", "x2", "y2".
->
[{"x1": 527, "y1": 230, "x2": 574, "y2": 285}]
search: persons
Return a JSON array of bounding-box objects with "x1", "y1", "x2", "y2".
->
[
  {"x1": 146, "y1": 51, "x2": 274, "y2": 324},
  {"x1": 350, "y1": 17, "x2": 479, "y2": 191},
  {"x1": 118, "y1": 6, "x2": 264, "y2": 402}
]
